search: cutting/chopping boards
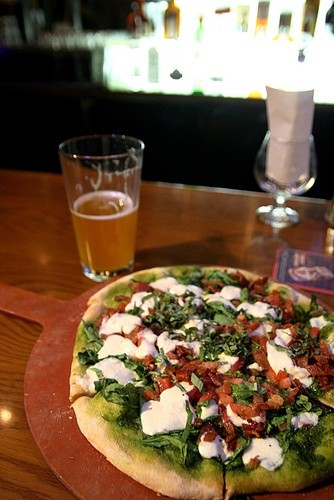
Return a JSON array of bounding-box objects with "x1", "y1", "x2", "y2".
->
[{"x1": 0, "y1": 264, "x2": 334, "y2": 500}]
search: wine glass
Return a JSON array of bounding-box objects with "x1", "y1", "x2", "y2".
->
[{"x1": 254, "y1": 128, "x2": 318, "y2": 229}]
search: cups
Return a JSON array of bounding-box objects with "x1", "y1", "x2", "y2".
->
[{"x1": 57, "y1": 133, "x2": 146, "y2": 284}]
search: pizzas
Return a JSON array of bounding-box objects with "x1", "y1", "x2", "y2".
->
[{"x1": 70, "y1": 265, "x2": 334, "y2": 498}]
